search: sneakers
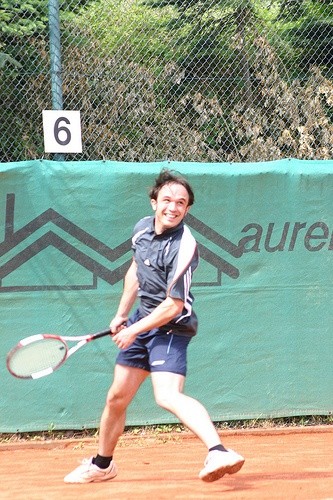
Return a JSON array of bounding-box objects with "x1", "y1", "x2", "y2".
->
[
  {"x1": 64, "y1": 456, "x2": 118, "y2": 484},
  {"x1": 199, "y1": 449, "x2": 245, "y2": 483}
]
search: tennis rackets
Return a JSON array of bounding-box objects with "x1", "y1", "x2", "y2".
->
[{"x1": 6, "y1": 321, "x2": 127, "y2": 380}]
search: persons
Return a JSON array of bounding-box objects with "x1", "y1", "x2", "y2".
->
[{"x1": 62, "y1": 169, "x2": 244, "y2": 484}]
